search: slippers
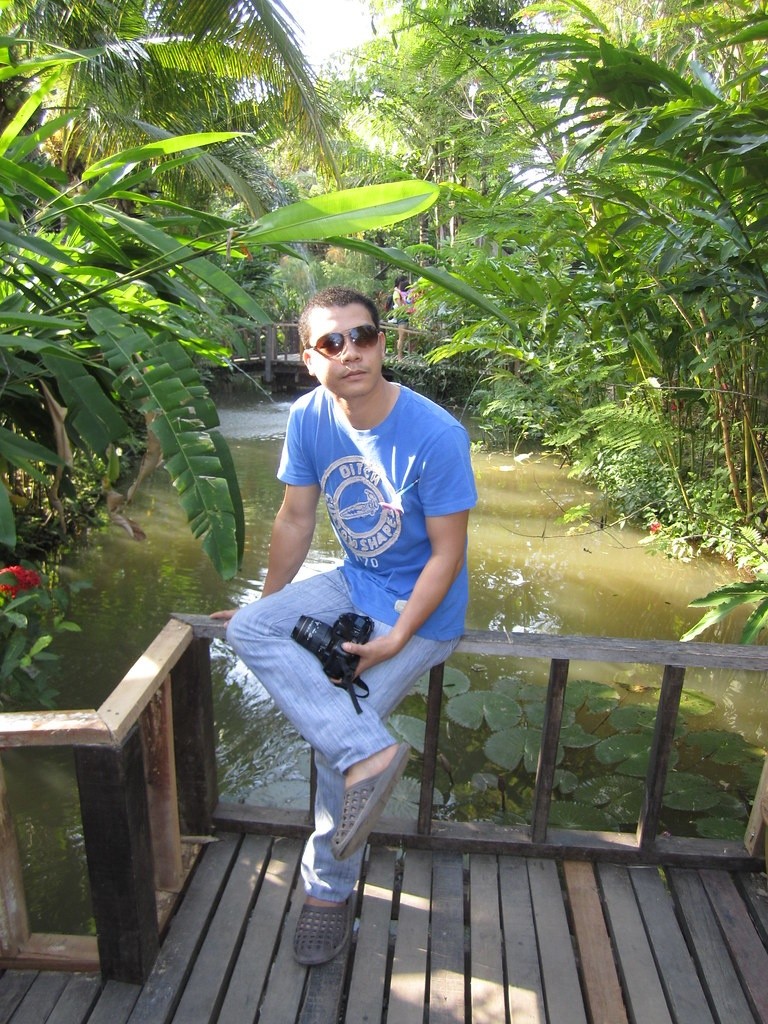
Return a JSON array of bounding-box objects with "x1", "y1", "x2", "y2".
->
[
  {"x1": 329, "y1": 742, "x2": 413, "y2": 858},
  {"x1": 291, "y1": 891, "x2": 352, "y2": 965}
]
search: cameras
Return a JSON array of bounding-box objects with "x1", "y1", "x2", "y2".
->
[{"x1": 290, "y1": 612, "x2": 375, "y2": 680}]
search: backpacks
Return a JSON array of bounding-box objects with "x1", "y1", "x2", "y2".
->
[{"x1": 384, "y1": 289, "x2": 396, "y2": 312}]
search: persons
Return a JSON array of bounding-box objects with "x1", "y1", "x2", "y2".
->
[
  {"x1": 206, "y1": 286, "x2": 479, "y2": 966},
  {"x1": 387, "y1": 275, "x2": 427, "y2": 362}
]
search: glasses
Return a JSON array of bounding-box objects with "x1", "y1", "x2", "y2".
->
[{"x1": 308, "y1": 322, "x2": 380, "y2": 357}]
